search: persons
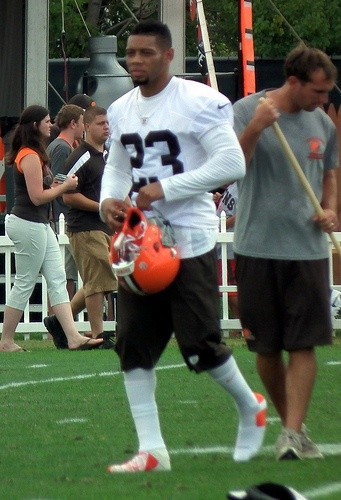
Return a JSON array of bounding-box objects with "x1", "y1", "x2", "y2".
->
[
  {"x1": 43, "y1": 106, "x2": 121, "y2": 349},
  {"x1": 0, "y1": 94, "x2": 115, "y2": 336},
  {"x1": 98, "y1": 21, "x2": 268, "y2": 472},
  {"x1": 213, "y1": 180, "x2": 244, "y2": 340},
  {"x1": 0, "y1": 105, "x2": 106, "y2": 352},
  {"x1": 230, "y1": 44, "x2": 341, "y2": 462}
]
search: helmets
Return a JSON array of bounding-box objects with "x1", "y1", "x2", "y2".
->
[{"x1": 108, "y1": 205, "x2": 180, "y2": 295}]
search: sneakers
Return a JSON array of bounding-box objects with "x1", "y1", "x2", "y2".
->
[
  {"x1": 292, "y1": 430, "x2": 324, "y2": 459},
  {"x1": 108, "y1": 447, "x2": 170, "y2": 472},
  {"x1": 233, "y1": 393, "x2": 267, "y2": 461},
  {"x1": 275, "y1": 429, "x2": 304, "y2": 462}
]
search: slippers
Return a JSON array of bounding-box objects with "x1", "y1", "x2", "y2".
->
[
  {"x1": 69, "y1": 337, "x2": 106, "y2": 351},
  {"x1": 13, "y1": 346, "x2": 31, "y2": 353}
]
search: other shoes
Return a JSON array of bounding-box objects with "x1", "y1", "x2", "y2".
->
[
  {"x1": 44, "y1": 315, "x2": 69, "y2": 349},
  {"x1": 90, "y1": 332, "x2": 116, "y2": 351}
]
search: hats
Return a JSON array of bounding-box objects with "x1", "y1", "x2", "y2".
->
[{"x1": 67, "y1": 93, "x2": 94, "y2": 109}]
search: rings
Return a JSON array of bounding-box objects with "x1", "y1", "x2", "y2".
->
[{"x1": 329, "y1": 221, "x2": 336, "y2": 228}]
